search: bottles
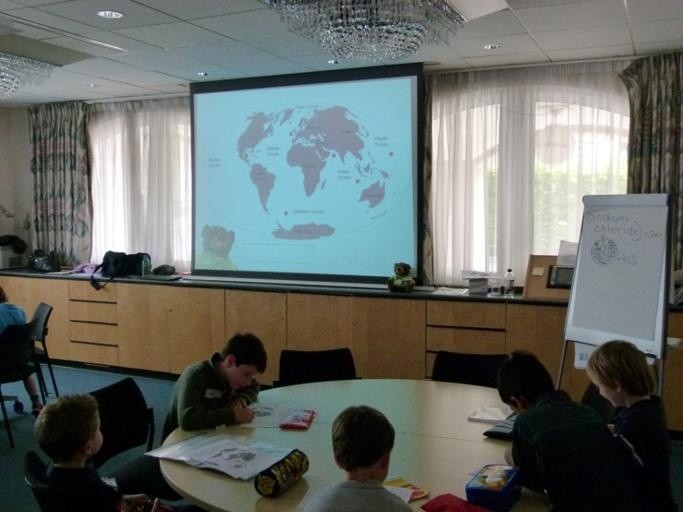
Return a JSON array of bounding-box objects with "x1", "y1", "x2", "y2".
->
[{"x1": 502, "y1": 267, "x2": 516, "y2": 299}]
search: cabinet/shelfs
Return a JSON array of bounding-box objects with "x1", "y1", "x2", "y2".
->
[
  {"x1": 32, "y1": 278, "x2": 71, "y2": 364},
  {"x1": 427, "y1": 299, "x2": 508, "y2": 380},
  {"x1": 2, "y1": 274, "x2": 32, "y2": 323},
  {"x1": 67, "y1": 280, "x2": 119, "y2": 370},
  {"x1": 506, "y1": 303, "x2": 599, "y2": 404},
  {"x1": 118, "y1": 282, "x2": 225, "y2": 378},
  {"x1": 226, "y1": 289, "x2": 285, "y2": 381},
  {"x1": 286, "y1": 293, "x2": 426, "y2": 378}
]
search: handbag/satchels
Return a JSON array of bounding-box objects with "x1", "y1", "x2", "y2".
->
[{"x1": 89, "y1": 250, "x2": 152, "y2": 291}]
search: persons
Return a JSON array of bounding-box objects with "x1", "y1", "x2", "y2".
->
[
  {"x1": 295, "y1": 405, "x2": 423, "y2": 511},
  {"x1": 35, "y1": 393, "x2": 207, "y2": 512},
  {"x1": 161, "y1": 333, "x2": 267, "y2": 446},
  {"x1": 586, "y1": 340, "x2": 672, "y2": 498},
  {"x1": 495, "y1": 351, "x2": 649, "y2": 512},
  {"x1": 0, "y1": 289, "x2": 40, "y2": 416}
]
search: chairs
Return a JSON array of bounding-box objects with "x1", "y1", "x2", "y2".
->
[
  {"x1": 431, "y1": 349, "x2": 510, "y2": 389},
  {"x1": 273, "y1": 345, "x2": 358, "y2": 388},
  {"x1": 0, "y1": 317, "x2": 47, "y2": 448},
  {"x1": 23, "y1": 451, "x2": 64, "y2": 511},
  {"x1": 83, "y1": 376, "x2": 184, "y2": 503}
]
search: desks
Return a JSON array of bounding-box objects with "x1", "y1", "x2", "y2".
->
[{"x1": 157, "y1": 377, "x2": 554, "y2": 511}]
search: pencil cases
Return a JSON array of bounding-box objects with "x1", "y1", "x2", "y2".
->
[{"x1": 465, "y1": 461, "x2": 522, "y2": 511}]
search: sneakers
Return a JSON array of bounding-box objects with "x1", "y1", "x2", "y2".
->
[{"x1": 31, "y1": 403, "x2": 42, "y2": 417}]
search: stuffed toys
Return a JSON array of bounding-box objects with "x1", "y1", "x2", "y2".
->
[{"x1": 387, "y1": 262, "x2": 415, "y2": 293}]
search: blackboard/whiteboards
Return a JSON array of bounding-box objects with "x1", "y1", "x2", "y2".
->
[{"x1": 566, "y1": 205, "x2": 670, "y2": 359}]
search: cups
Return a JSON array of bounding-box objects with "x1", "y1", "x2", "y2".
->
[{"x1": 490, "y1": 278, "x2": 502, "y2": 296}]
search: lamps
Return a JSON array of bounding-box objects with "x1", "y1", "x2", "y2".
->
[
  {"x1": 264, "y1": 1, "x2": 467, "y2": 62},
  {"x1": 1, "y1": 51, "x2": 62, "y2": 99}
]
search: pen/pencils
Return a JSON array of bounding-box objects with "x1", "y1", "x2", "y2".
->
[{"x1": 239, "y1": 397, "x2": 246, "y2": 408}]
